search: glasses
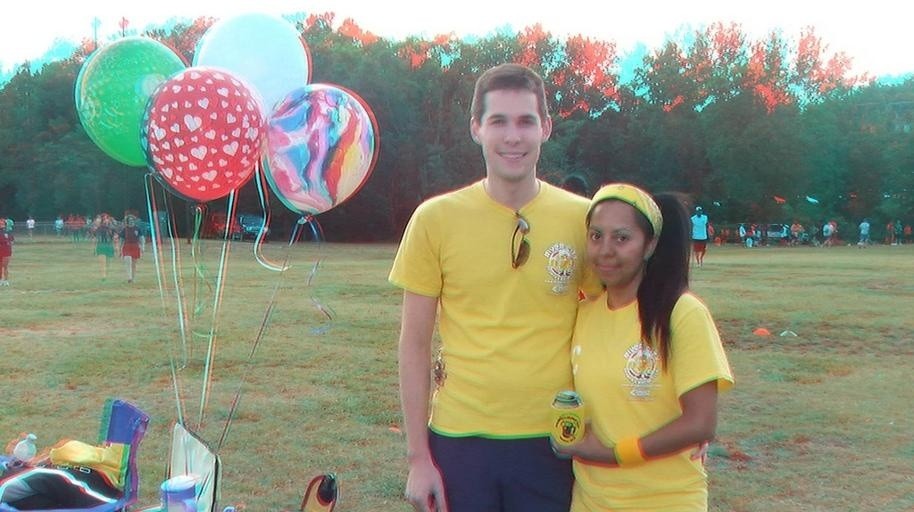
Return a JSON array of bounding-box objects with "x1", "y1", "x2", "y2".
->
[{"x1": 512, "y1": 211, "x2": 531, "y2": 270}]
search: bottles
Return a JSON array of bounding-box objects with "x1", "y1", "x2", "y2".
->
[{"x1": 9, "y1": 432, "x2": 39, "y2": 473}]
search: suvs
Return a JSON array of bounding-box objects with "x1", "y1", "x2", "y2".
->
[{"x1": 239, "y1": 215, "x2": 267, "y2": 241}]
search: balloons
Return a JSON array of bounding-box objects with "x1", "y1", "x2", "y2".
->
[
  {"x1": 140, "y1": 66, "x2": 263, "y2": 204},
  {"x1": 193, "y1": 13, "x2": 311, "y2": 122},
  {"x1": 75, "y1": 36, "x2": 192, "y2": 168},
  {"x1": 260, "y1": 83, "x2": 380, "y2": 218}
]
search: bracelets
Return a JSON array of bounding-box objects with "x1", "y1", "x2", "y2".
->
[{"x1": 615, "y1": 435, "x2": 644, "y2": 469}]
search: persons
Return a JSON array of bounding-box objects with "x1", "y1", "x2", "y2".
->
[
  {"x1": 560, "y1": 175, "x2": 588, "y2": 197},
  {"x1": 1, "y1": 214, "x2": 145, "y2": 287},
  {"x1": 388, "y1": 64, "x2": 712, "y2": 512},
  {"x1": 433, "y1": 183, "x2": 733, "y2": 512},
  {"x1": 858, "y1": 218, "x2": 911, "y2": 247},
  {"x1": 689, "y1": 206, "x2": 709, "y2": 268},
  {"x1": 708, "y1": 220, "x2": 838, "y2": 248}
]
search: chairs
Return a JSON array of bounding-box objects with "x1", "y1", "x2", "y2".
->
[
  {"x1": 300, "y1": 472, "x2": 338, "y2": 512},
  {"x1": 1, "y1": 401, "x2": 149, "y2": 512},
  {"x1": 136, "y1": 425, "x2": 221, "y2": 512}
]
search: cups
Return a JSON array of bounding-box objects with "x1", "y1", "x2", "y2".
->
[{"x1": 160, "y1": 474, "x2": 198, "y2": 511}]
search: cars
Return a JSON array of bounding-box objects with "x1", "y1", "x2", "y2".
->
[{"x1": 199, "y1": 213, "x2": 241, "y2": 238}]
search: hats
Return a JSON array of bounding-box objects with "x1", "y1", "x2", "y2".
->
[{"x1": 695, "y1": 206, "x2": 703, "y2": 211}]
